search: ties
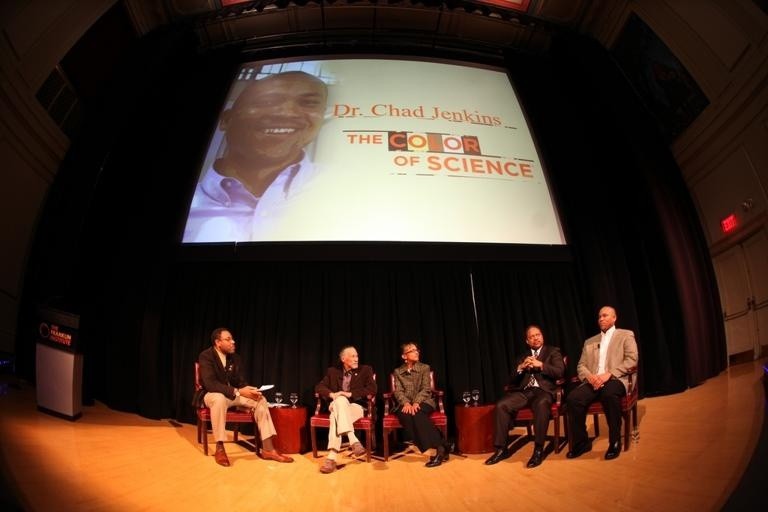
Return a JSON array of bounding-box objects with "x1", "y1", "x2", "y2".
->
[{"x1": 526, "y1": 351, "x2": 538, "y2": 388}]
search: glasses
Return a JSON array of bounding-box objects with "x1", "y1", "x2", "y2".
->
[{"x1": 405, "y1": 348, "x2": 420, "y2": 354}]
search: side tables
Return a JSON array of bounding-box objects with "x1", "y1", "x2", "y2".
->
[
  {"x1": 259, "y1": 404, "x2": 311, "y2": 454},
  {"x1": 454, "y1": 403, "x2": 498, "y2": 454}
]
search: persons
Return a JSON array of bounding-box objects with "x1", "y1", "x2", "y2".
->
[
  {"x1": 566, "y1": 305, "x2": 640, "y2": 460},
  {"x1": 392, "y1": 340, "x2": 451, "y2": 469},
  {"x1": 482, "y1": 323, "x2": 566, "y2": 469},
  {"x1": 181, "y1": 69, "x2": 329, "y2": 245},
  {"x1": 197, "y1": 327, "x2": 294, "y2": 468},
  {"x1": 315, "y1": 345, "x2": 378, "y2": 474}
]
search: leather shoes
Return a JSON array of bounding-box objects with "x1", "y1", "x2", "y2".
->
[
  {"x1": 319, "y1": 458, "x2": 337, "y2": 474},
  {"x1": 605, "y1": 439, "x2": 622, "y2": 460},
  {"x1": 425, "y1": 452, "x2": 449, "y2": 467},
  {"x1": 526, "y1": 448, "x2": 544, "y2": 468},
  {"x1": 261, "y1": 449, "x2": 294, "y2": 463},
  {"x1": 484, "y1": 447, "x2": 509, "y2": 465},
  {"x1": 352, "y1": 441, "x2": 368, "y2": 458},
  {"x1": 215, "y1": 448, "x2": 230, "y2": 467},
  {"x1": 566, "y1": 443, "x2": 592, "y2": 458}
]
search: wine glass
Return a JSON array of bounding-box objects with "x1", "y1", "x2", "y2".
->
[
  {"x1": 290, "y1": 393, "x2": 298, "y2": 410},
  {"x1": 472, "y1": 390, "x2": 480, "y2": 406},
  {"x1": 462, "y1": 392, "x2": 471, "y2": 407},
  {"x1": 275, "y1": 393, "x2": 282, "y2": 409}
]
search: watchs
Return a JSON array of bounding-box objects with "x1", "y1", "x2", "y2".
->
[{"x1": 235, "y1": 388, "x2": 240, "y2": 398}]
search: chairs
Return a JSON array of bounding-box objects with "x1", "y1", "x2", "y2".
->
[
  {"x1": 566, "y1": 366, "x2": 639, "y2": 451},
  {"x1": 194, "y1": 362, "x2": 260, "y2": 456},
  {"x1": 383, "y1": 371, "x2": 447, "y2": 462},
  {"x1": 503, "y1": 356, "x2": 566, "y2": 453},
  {"x1": 310, "y1": 374, "x2": 377, "y2": 463}
]
